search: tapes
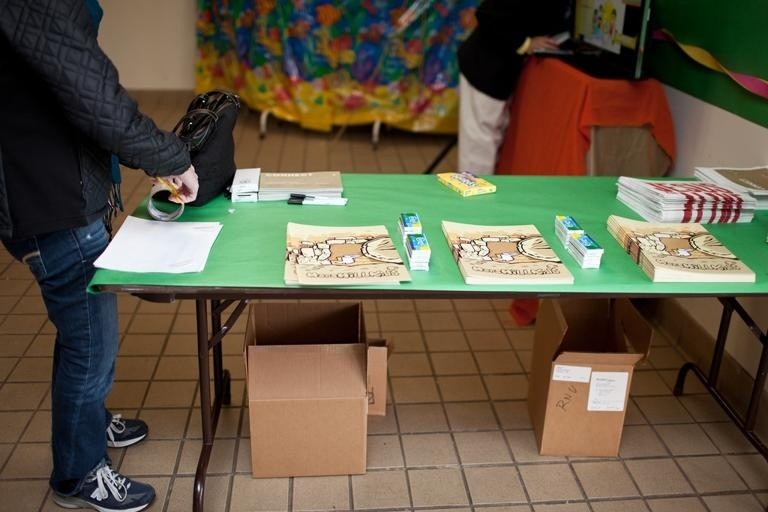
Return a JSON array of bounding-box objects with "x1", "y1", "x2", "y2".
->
[{"x1": 147, "y1": 183, "x2": 184, "y2": 221}]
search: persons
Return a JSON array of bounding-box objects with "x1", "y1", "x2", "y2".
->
[
  {"x1": 0, "y1": 0, "x2": 200, "y2": 512},
  {"x1": 457, "y1": 0, "x2": 569, "y2": 174}
]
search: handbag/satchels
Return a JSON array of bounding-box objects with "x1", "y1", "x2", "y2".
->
[{"x1": 150, "y1": 89, "x2": 242, "y2": 208}]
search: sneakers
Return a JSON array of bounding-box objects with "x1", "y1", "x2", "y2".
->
[
  {"x1": 104, "y1": 413, "x2": 149, "y2": 448},
  {"x1": 51, "y1": 464, "x2": 155, "y2": 512}
]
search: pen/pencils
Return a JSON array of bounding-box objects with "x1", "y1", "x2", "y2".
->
[{"x1": 288, "y1": 193, "x2": 349, "y2": 206}]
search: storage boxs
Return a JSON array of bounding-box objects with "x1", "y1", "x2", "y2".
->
[
  {"x1": 528, "y1": 297, "x2": 654, "y2": 458},
  {"x1": 243, "y1": 298, "x2": 395, "y2": 478}
]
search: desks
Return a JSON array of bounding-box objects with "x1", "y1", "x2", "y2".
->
[
  {"x1": 87, "y1": 172, "x2": 768, "y2": 512},
  {"x1": 500, "y1": 53, "x2": 676, "y2": 177}
]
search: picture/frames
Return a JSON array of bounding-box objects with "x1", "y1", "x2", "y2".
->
[{"x1": 558, "y1": 0, "x2": 650, "y2": 78}]
